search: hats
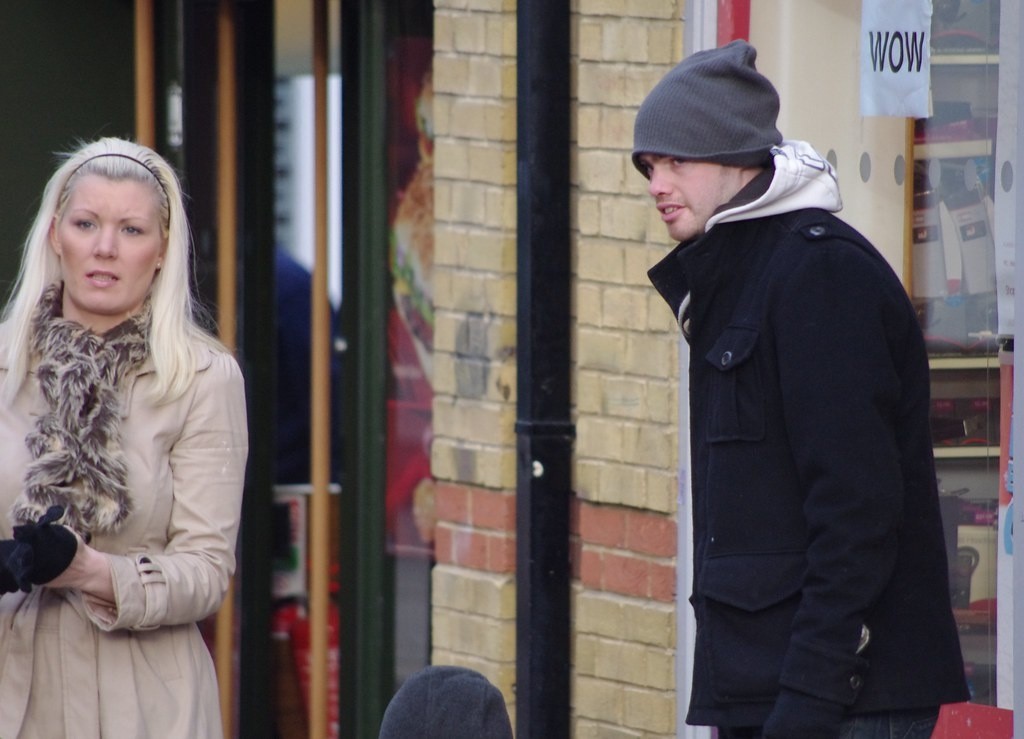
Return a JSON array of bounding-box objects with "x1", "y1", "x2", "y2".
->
[
  {"x1": 632, "y1": 39, "x2": 783, "y2": 181},
  {"x1": 379, "y1": 666, "x2": 514, "y2": 739}
]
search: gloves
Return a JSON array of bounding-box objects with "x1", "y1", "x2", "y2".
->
[
  {"x1": 0, "y1": 539, "x2": 32, "y2": 594},
  {"x1": 13, "y1": 505, "x2": 77, "y2": 584},
  {"x1": 762, "y1": 687, "x2": 843, "y2": 739}
]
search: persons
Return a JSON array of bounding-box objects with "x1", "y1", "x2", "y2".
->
[
  {"x1": 380, "y1": 665, "x2": 514, "y2": 739},
  {"x1": 0, "y1": 136, "x2": 248, "y2": 739},
  {"x1": 629, "y1": 39, "x2": 973, "y2": 739}
]
